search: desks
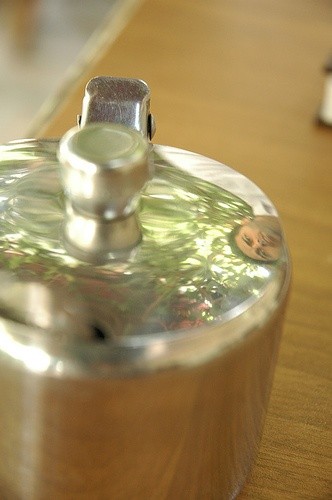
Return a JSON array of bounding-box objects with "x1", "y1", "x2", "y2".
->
[{"x1": 22, "y1": 2, "x2": 330, "y2": 499}]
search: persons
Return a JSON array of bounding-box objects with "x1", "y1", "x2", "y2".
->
[{"x1": 227, "y1": 199, "x2": 287, "y2": 267}]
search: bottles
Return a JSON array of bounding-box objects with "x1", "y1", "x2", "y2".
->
[{"x1": 0, "y1": 75, "x2": 290, "y2": 499}]
